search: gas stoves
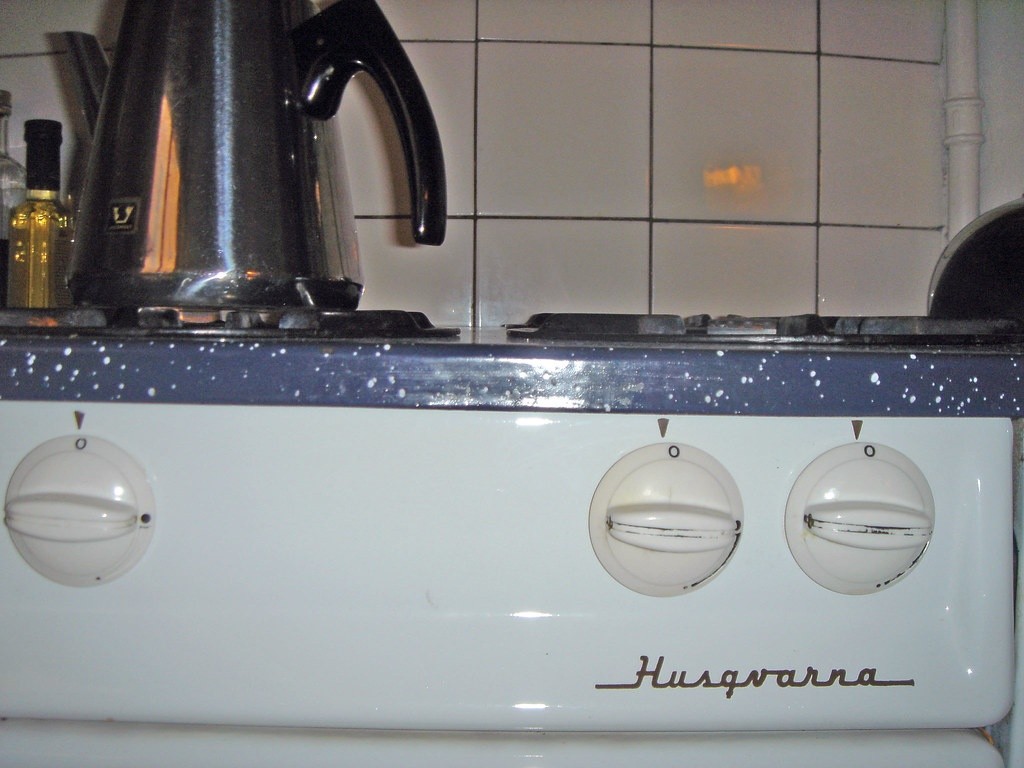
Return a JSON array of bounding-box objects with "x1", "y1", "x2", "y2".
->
[{"x1": 0, "y1": 303, "x2": 1024, "y2": 733}]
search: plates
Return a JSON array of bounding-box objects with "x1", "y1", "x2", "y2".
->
[{"x1": 925, "y1": 194, "x2": 1024, "y2": 319}]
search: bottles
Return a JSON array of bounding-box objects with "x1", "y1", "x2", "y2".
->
[
  {"x1": 7, "y1": 118, "x2": 75, "y2": 309},
  {"x1": 0, "y1": 88, "x2": 26, "y2": 242}
]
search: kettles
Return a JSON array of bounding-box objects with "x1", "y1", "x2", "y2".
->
[{"x1": 63, "y1": 0, "x2": 452, "y2": 306}]
